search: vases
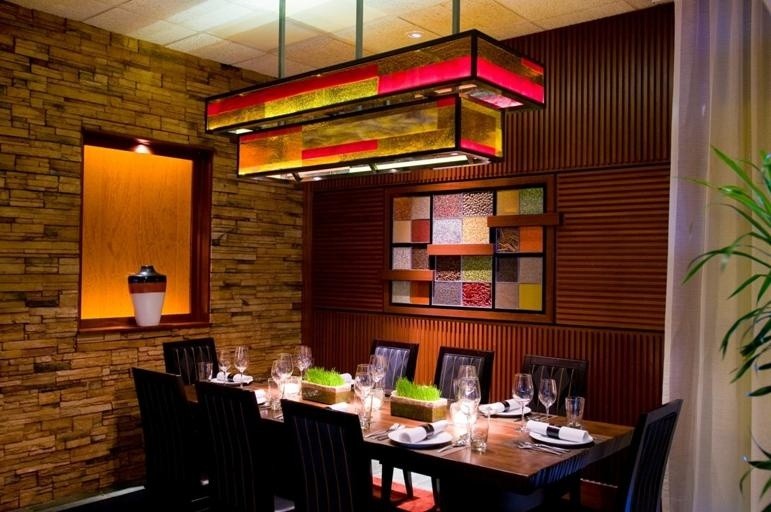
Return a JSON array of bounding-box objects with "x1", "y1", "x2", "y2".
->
[{"x1": 128, "y1": 265, "x2": 167, "y2": 325}]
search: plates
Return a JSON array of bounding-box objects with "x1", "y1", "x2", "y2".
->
[
  {"x1": 492, "y1": 405, "x2": 532, "y2": 417},
  {"x1": 390, "y1": 428, "x2": 454, "y2": 447},
  {"x1": 527, "y1": 429, "x2": 594, "y2": 446}
]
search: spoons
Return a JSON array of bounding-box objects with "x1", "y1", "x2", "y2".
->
[
  {"x1": 437, "y1": 432, "x2": 468, "y2": 454},
  {"x1": 366, "y1": 422, "x2": 399, "y2": 440}
]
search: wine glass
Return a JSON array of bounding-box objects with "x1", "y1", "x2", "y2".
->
[
  {"x1": 538, "y1": 379, "x2": 558, "y2": 422},
  {"x1": 513, "y1": 373, "x2": 534, "y2": 426},
  {"x1": 218, "y1": 351, "x2": 232, "y2": 384},
  {"x1": 234, "y1": 346, "x2": 249, "y2": 388}
]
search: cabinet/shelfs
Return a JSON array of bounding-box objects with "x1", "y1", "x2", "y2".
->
[{"x1": 384, "y1": 210, "x2": 562, "y2": 284}]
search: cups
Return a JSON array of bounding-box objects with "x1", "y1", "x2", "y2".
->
[
  {"x1": 565, "y1": 396, "x2": 586, "y2": 427},
  {"x1": 294, "y1": 345, "x2": 312, "y2": 373},
  {"x1": 270, "y1": 353, "x2": 295, "y2": 385},
  {"x1": 353, "y1": 354, "x2": 388, "y2": 432},
  {"x1": 467, "y1": 408, "x2": 490, "y2": 453},
  {"x1": 453, "y1": 363, "x2": 482, "y2": 423},
  {"x1": 267, "y1": 379, "x2": 285, "y2": 411},
  {"x1": 198, "y1": 362, "x2": 214, "y2": 382}
]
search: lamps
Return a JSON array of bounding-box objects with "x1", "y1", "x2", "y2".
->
[{"x1": 204, "y1": 1, "x2": 544, "y2": 194}]
